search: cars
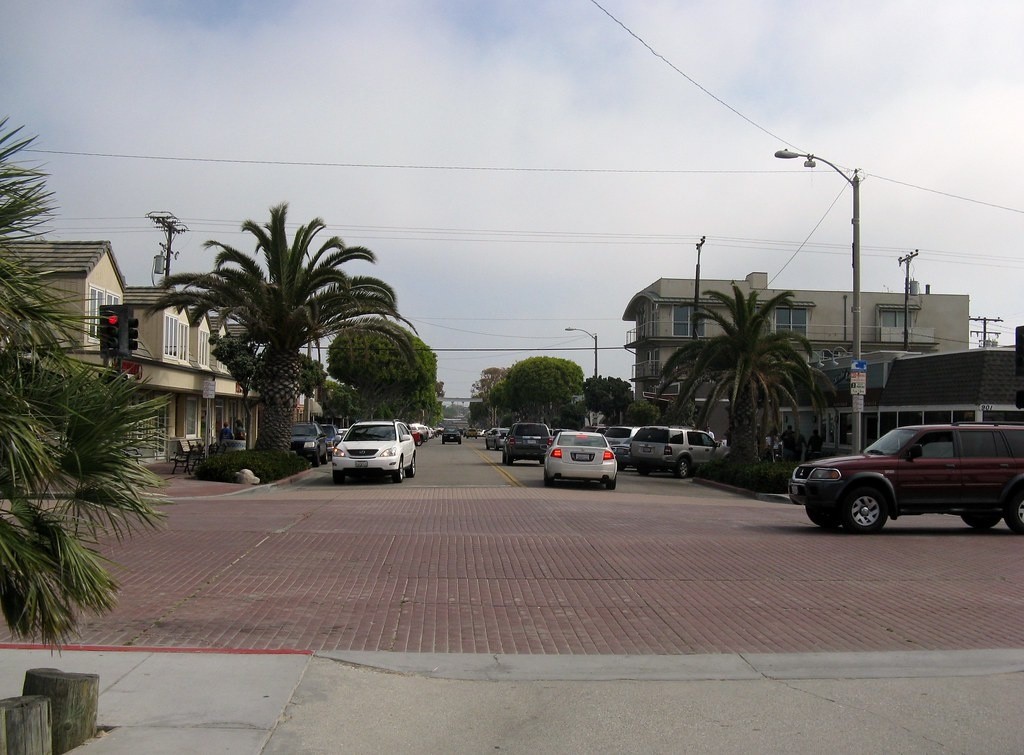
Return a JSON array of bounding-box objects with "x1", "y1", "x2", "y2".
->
[
  {"x1": 335, "y1": 427, "x2": 349, "y2": 440},
  {"x1": 466, "y1": 428, "x2": 477, "y2": 439},
  {"x1": 540, "y1": 431, "x2": 618, "y2": 489},
  {"x1": 458, "y1": 427, "x2": 486, "y2": 436},
  {"x1": 408, "y1": 423, "x2": 444, "y2": 446},
  {"x1": 442, "y1": 428, "x2": 462, "y2": 444},
  {"x1": 485, "y1": 428, "x2": 509, "y2": 450},
  {"x1": 549, "y1": 428, "x2": 575, "y2": 442},
  {"x1": 580, "y1": 425, "x2": 611, "y2": 436}
]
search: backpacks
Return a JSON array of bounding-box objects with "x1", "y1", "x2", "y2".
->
[
  {"x1": 238, "y1": 432, "x2": 247, "y2": 440},
  {"x1": 222, "y1": 431, "x2": 229, "y2": 439}
]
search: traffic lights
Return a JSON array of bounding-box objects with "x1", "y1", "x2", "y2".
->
[
  {"x1": 122, "y1": 303, "x2": 138, "y2": 360},
  {"x1": 1014, "y1": 326, "x2": 1024, "y2": 377},
  {"x1": 1015, "y1": 390, "x2": 1024, "y2": 409},
  {"x1": 100, "y1": 305, "x2": 121, "y2": 357}
]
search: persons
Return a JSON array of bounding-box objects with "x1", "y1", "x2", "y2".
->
[
  {"x1": 706, "y1": 426, "x2": 714, "y2": 440},
  {"x1": 765, "y1": 425, "x2": 807, "y2": 464},
  {"x1": 219, "y1": 422, "x2": 233, "y2": 441},
  {"x1": 806, "y1": 429, "x2": 823, "y2": 453},
  {"x1": 234, "y1": 420, "x2": 245, "y2": 440}
]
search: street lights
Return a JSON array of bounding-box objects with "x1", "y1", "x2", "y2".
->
[
  {"x1": 774, "y1": 149, "x2": 863, "y2": 457},
  {"x1": 565, "y1": 328, "x2": 599, "y2": 381}
]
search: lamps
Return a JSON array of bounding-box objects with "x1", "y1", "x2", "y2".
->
[
  {"x1": 812, "y1": 415, "x2": 817, "y2": 423},
  {"x1": 817, "y1": 349, "x2": 837, "y2": 368},
  {"x1": 831, "y1": 346, "x2": 849, "y2": 367}
]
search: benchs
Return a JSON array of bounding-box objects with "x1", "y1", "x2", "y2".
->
[{"x1": 170, "y1": 440, "x2": 228, "y2": 476}]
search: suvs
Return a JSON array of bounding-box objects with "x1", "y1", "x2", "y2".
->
[
  {"x1": 289, "y1": 421, "x2": 329, "y2": 467},
  {"x1": 321, "y1": 424, "x2": 339, "y2": 459},
  {"x1": 604, "y1": 425, "x2": 642, "y2": 469},
  {"x1": 330, "y1": 419, "x2": 416, "y2": 483},
  {"x1": 786, "y1": 421, "x2": 1024, "y2": 534},
  {"x1": 501, "y1": 423, "x2": 553, "y2": 464},
  {"x1": 629, "y1": 426, "x2": 735, "y2": 478}
]
